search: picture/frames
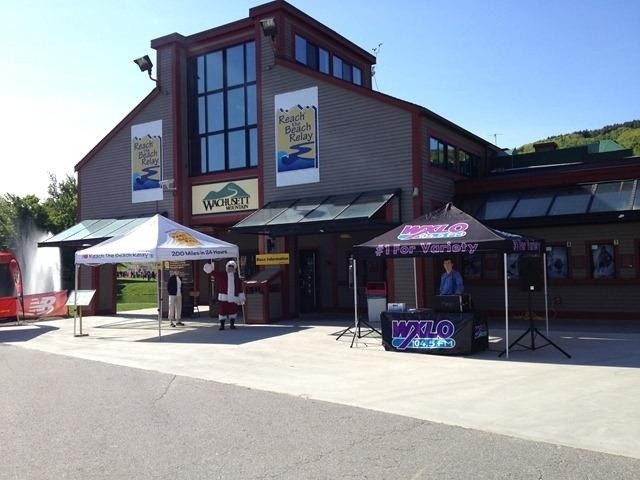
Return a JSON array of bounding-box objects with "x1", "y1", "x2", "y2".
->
[
  {"x1": 457, "y1": 253, "x2": 486, "y2": 288},
  {"x1": 507, "y1": 253, "x2": 520, "y2": 288},
  {"x1": 584, "y1": 239, "x2": 621, "y2": 285},
  {"x1": 546, "y1": 240, "x2": 574, "y2": 287}
]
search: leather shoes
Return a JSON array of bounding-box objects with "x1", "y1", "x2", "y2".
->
[{"x1": 171, "y1": 321, "x2": 185, "y2": 327}]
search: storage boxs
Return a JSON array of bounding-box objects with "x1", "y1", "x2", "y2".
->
[
  {"x1": 433, "y1": 292, "x2": 472, "y2": 313},
  {"x1": 387, "y1": 302, "x2": 407, "y2": 313}
]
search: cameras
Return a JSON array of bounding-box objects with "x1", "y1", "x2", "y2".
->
[{"x1": 527, "y1": 279, "x2": 536, "y2": 291}]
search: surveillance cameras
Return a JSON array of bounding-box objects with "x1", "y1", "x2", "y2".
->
[{"x1": 158, "y1": 179, "x2": 175, "y2": 186}]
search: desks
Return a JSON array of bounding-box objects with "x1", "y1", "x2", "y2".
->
[{"x1": 379, "y1": 308, "x2": 489, "y2": 356}]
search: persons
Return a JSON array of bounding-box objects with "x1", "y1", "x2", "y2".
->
[
  {"x1": 439, "y1": 258, "x2": 465, "y2": 295},
  {"x1": 168, "y1": 268, "x2": 184, "y2": 327},
  {"x1": 203, "y1": 261, "x2": 246, "y2": 331}
]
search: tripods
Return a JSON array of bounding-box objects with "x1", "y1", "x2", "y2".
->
[
  {"x1": 498, "y1": 294, "x2": 572, "y2": 359},
  {"x1": 336, "y1": 261, "x2": 382, "y2": 348}
]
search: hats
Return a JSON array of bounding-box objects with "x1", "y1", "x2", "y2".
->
[{"x1": 225, "y1": 260, "x2": 237, "y2": 271}]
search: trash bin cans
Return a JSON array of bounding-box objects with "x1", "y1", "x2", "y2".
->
[{"x1": 365, "y1": 280, "x2": 389, "y2": 325}]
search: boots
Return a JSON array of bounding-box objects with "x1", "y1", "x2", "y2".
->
[{"x1": 218, "y1": 318, "x2": 236, "y2": 331}]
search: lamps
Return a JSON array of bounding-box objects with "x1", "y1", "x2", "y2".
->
[
  {"x1": 259, "y1": 17, "x2": 278, "y2": 42},
  {"x1": 133, "y1": 55, "x2": 158, "y2": 82}
]
